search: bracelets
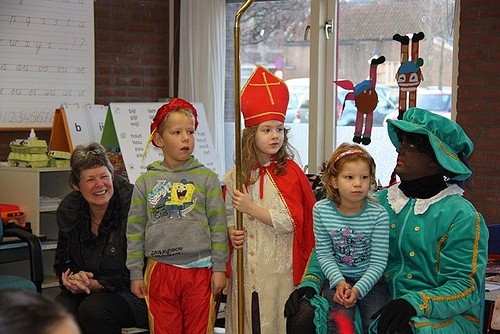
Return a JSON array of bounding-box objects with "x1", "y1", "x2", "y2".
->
[{"x1": 99, "y1": 285, "x2": 105, "y2": 291}]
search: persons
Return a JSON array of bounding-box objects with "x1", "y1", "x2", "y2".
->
[
  {"x1": 283, "y1": 105, "x2": 489, "y2": 334},
  {"x1": 313, "y1": 142, "x2": 394, "y2": 334},
  {"x1": 224, "y1": 64, "x2": 316, "y2": 334},
  {"x1": 51, "y1": 141, "x2": 149, "y2": 334},
  {"x1": 0, "y1": 288, "x2": 81, "y2": 334},
  {"x1": 125, "y1": 98, "x2": 230, "y2": 334}
]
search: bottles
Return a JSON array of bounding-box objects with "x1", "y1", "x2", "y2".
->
[{"x1": 28, "y1": 129, "x2": 37, "y2": 139}]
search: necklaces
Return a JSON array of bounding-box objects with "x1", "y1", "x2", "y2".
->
[{"x1": 90, "y1": 217, "x2": 100, "y2": 226}]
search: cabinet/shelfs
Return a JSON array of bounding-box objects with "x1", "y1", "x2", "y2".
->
[{"x1": 1, "y1": 165, "x2": 90, "y2": 288}]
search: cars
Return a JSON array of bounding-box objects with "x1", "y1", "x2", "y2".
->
[{"x1": 281, "y1": 78, "x2": 452, "y2": 127}]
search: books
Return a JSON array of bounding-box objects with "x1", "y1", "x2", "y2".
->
[
  {"x1": 484, "y1": 258, "x2": 500, "y2": 301},
  {"x1": 39, "y1": 195, "x2": 62, "y2": 206}
]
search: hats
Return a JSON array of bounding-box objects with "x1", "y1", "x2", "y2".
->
[
  {"x1": 386, "y1": 108, "x2": 474, "y2": 181},
  {"x1": 241, "y1": 65, "x2": 290, "y2": 127}
]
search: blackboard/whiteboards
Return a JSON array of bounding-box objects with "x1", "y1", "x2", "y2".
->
[{"x1": 0, "y1": 1, "x2": 95, "y2": 130}]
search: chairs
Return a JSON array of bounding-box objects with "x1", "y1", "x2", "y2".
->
[{"x1": 1, "y1": 218, "x2": 44, "y2": 295}]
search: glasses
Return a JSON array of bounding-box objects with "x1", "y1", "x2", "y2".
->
[{"x1": 71, "y1": 148, "x2": 111, "y2": 173}]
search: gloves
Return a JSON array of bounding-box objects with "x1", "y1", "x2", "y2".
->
[
  {"x1": 284, "y1": 286, "x2": 315, "y2": 317},
  {"x1": 370, "y1": 299, "x2": 416, "y2": 334}
]
